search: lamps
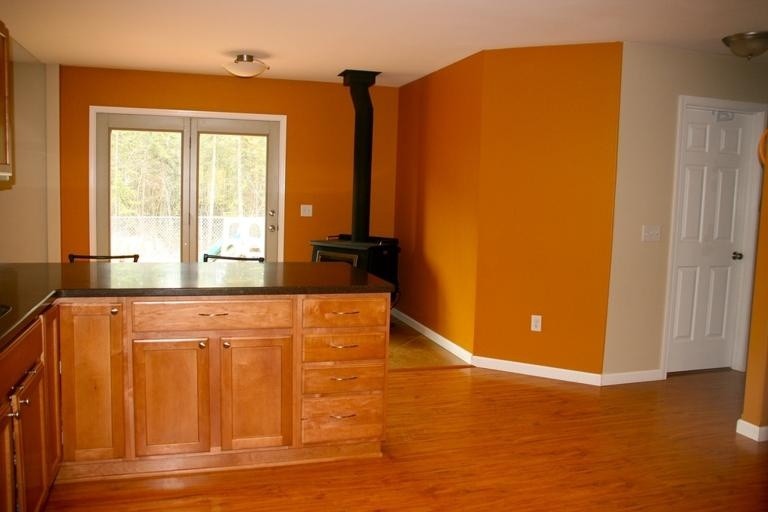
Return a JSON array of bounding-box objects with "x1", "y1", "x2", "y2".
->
[
  {"x1": 722, "y1": 32, "x2": 768, "y2": 60},
  {"x1": 222, "y1": 53, "x2": 270, "y2": 78}
]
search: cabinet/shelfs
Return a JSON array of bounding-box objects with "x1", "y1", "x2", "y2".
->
[
  {"x1": 296, "y1": 293, "x2": 391, "y2": 466},
  {"x1": 0, "y1": 318, "x2": 45, "y2": 511},
  {"x1": 56, "y1": 297, "x2": 125, "y2": 485},
  {"x1": 124, "y1": 294, "x2": 297, "y2": 479},
  {"x1": 38, "y1": 304, "x2": 62, "y2": 497}
]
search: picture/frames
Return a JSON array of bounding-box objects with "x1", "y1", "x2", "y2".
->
[{"x1": 0, "y1": 21, "x2": 16, "y2": 191}]
